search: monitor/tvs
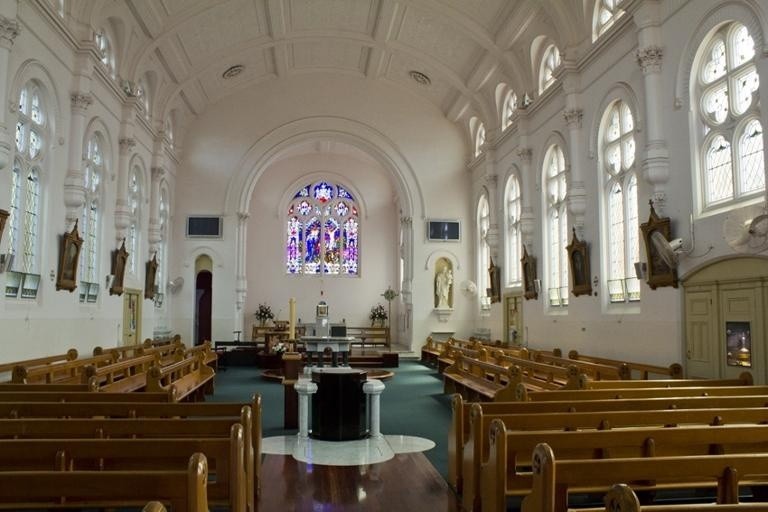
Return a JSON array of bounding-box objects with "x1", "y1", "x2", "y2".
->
[
  {"x1": 428, "y1": 220, "x2": 461, "y2": 241},
  {"x1": 187, "y1": 215, "x2": 221, "y2": 238}
]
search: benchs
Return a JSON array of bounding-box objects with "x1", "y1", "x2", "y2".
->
[
  {"x1": 0, "y1": 348, "x2": 78, "y2": 374},
  {"x1": 447, "y1": 393, "x2": 767, "y2": 493},
  {"x1": 448, "y1": 337, "x2": 477, "y2": 350},
  {"x1": 139, "y1": 341, "x2": 186, "y2": 353},
  {"x1": 145, "y1": 335, "x2": 182, "y2": 345},
  {"x1": 0, "y1": 384, "x2": 178, "y2": 402},
  {"x1": 420, "y1": 335, "x2": 451, "y2": 366},
  {"x1": 439, "y1": 344, "x2": 486, "y2": 372},
  {"x1": 494, "y1": 348, "x2": 581, "y2": 391},
  {"x1": 516, "y1": 383, "x2": 768, "y2": 400},
  {"x1": 94, "y1": 344, "x2": 144, "y2": 359},
  {"x1": 82, "y1": 351, "x2": 163, "y2": 393},
  {"x1": 12, "y1": 350, "x2": 119, "y2": 384},
  {"x1": 444, "y1": 351, "x2": 520, "y2": 403},
  {"x1": 462, "y1": 403, "x2": 768, "y2": 512},
  {"x1": 0, "y1": 451, "x2": 209, "y2": 512},
  {"x1": 480, "y1": 418, "x2": 766, "y2": 512},
  {"x1": 146, "y1": 353, "x2": 216, "y2": 402},
  {"x1": 2, "y1": 424, "x2": 248, "y2": 511},
  {"x1": 519, "y1": 347, "x2": 631, "y2": 380},
  {"x1": 569, "y1": 351, "x2": 682, "y2": 379},
  {"x1": 476, "y1": 340, "x2": 531, "y2": 360},
  {"x1": 520, "y1": 443, "x2": 767, "y2": 512},
  {"x1": 603, "y1": 482, "x2": 642, "y2": 512},
  {"x1": 174, "y1": 341, "x2": 218, "y2": 370},
  {"x1": 580, "y1": 372, "x2": 755, "y2": 388}
]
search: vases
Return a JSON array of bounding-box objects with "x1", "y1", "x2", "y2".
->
[
  {"x1": 259, "y1": 317, "x2": 267, "y2": 327},
  {"x1": 374, "y1": 317, "x2": 383, "y2": 327}
]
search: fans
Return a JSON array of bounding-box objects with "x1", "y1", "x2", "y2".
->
[
  {"x1": 650, "y1": 232, "x2": 689, "y2": 269},
  {"x1": 459, "y1": 280, "x2": 478, "y2": 306},
  {"x1": 168, "y1": 276, "x2": 186, "y2": 296},
  {"x1": 721, "y1": 213, "x2": 767, "y2": 254}
]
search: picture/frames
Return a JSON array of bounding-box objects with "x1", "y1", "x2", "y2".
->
[
  {"x1": 147, "y1": 251, "x2": 158, "y2": 299},
  {"x1": 565, "y1": 227, "x2": 592, "y2": 296},
  {"x1": 640, "y1": 198, "x2": 677, "y2": 291},
  {"x1": 0, "y1": 210, "x2": 8, "y2": 241},
  {"x1": 487, "y1": 255, "x2": 504, "y2": 304},
  {"x1": 521, "y1": 244, "x2": 539, "y2": 300},
  {"x1": 109, "y1": 237, "x2": 129, "y2": 296},
  {"x1": 56, "y1": 217, "x2": 83, "y2": 292}
]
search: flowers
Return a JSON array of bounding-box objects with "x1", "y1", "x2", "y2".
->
[
  {"x1": 370, "y1": 305, "x2": 388, "y2": 320},
  {"x1": 255, "y1": 303, "x2": 275, "y2": 319}
]
